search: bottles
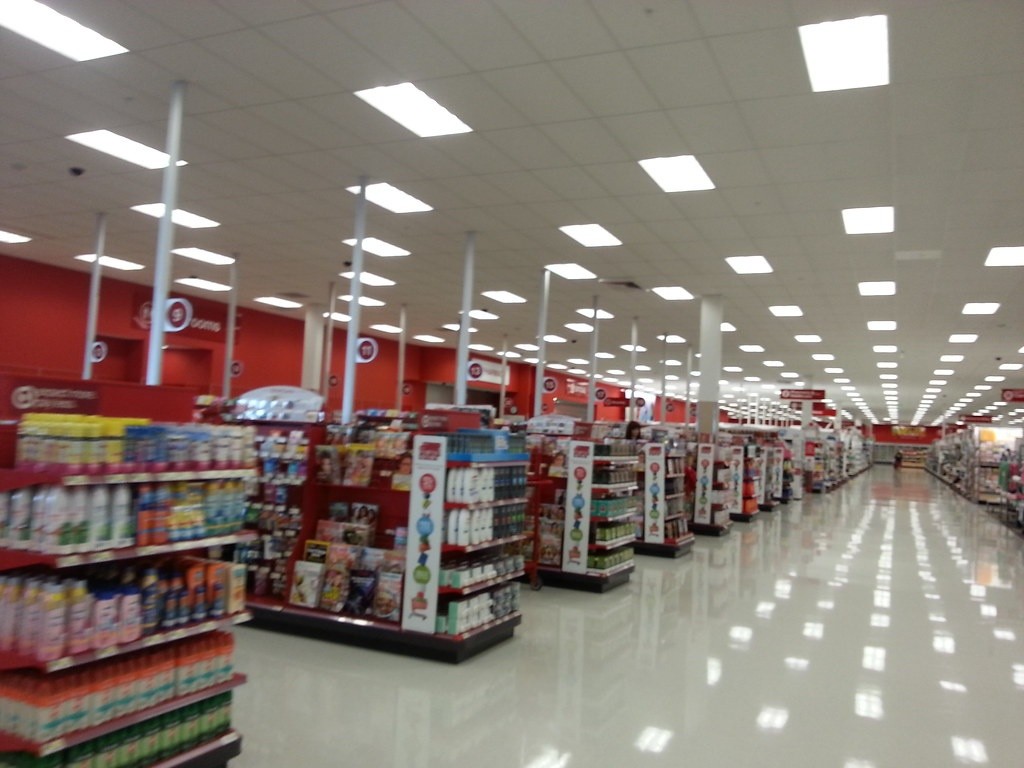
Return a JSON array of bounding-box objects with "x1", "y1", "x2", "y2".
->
[
  {"x1": 0, "y1": 690, "x2": 233, "y2": 768},
  {"x1": 16, "y1": 413, "x2": 257, "y2": 473},
  {"x1": 0, "y1": 483, "x2": 135, "y2": 555},
  {"x1": 136, "y1": 480, "x2": 248, "y2": 546},
  {"x1": 1, "y1": 632, "x2": 247, "y2": 744},
  {"x1": 0, "y1": 575, "x2": 91, "y2": 661}
]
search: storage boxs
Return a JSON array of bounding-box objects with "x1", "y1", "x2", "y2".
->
[{"x1": 288, "y1": 431, "x2": 690, "y2": 636}]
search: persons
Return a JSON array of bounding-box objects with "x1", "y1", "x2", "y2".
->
[
  {"x1": 538, "y1": 491, "x2": 566, "y2": 565},
  {"x1": 549, "y1": 452, "x2": 566, "y2": 477},
  {"x1": 316, "y1": 450, "x2": 335, "y2": 473},
  {"x1": 396, "y1": 452, "x2": 412, "y2": 475},
  {"x1": 349, "y1": 505, "x2": 376, "y2": 525},
  {"x1": 893, "y1": 449, "x2": 903, "y2": 469},
  {"x1": 344, "y1": 448, "x2": 374, "y2": 486},
  {"x1": 625, "y1": 421, "x2": 641, "y2": 439},
  {"x1": 321, "y1": 568, "x2": 349, "y2": 602}
]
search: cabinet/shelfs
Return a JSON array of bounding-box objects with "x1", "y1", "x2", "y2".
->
[
  {"x1": 494, "y1": 420, "x2": 641, "y2": 593},
  {"x1": 0, "y1": 367, "x2": 250, "y2": 768},
  {"x1": 198, "y1": 397, "x2": 531, "y2": 664},
  {"x1": 634, "y1": 428, "x2": 874, "y2": 559},
  {"x1": 925, "y1": 424, "x2": 1024, "y2": 537}
]
